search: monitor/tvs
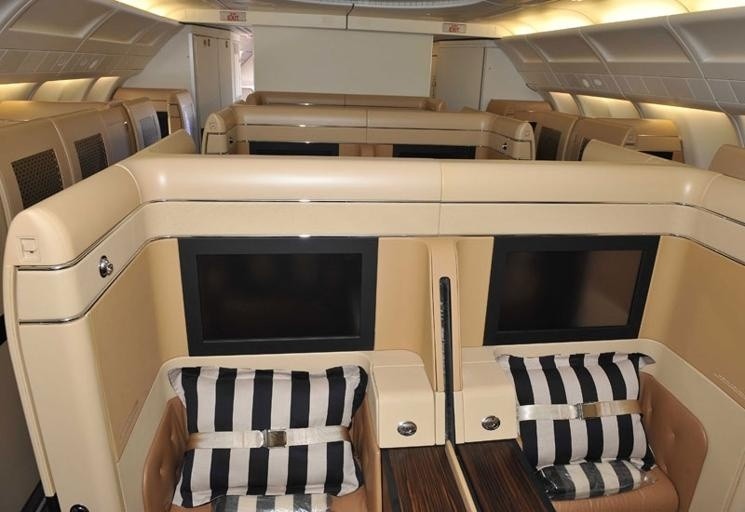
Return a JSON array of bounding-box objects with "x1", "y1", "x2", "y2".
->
[
  {"x1": 249, "y1": 141, "x2": 338, "y2": 156},
  {"x1": 392, "y1": 144, "x2": 475, "y2": 160},
  {"x1": 177, "y1": 237, "x2": 378, "y2": 357},
  {"x1": 483, "y1": 236, "x2": 661, "y2": 345},
  {"x1": 640, "y1": 151, "x2": 672, "y2": 160},
  {"x1": 156, "y1": 111, "x2": 169, "y2": 138}
]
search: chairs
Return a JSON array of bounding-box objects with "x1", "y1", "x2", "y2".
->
[{"x1": 0, "y1": 73, "x2": 745, "y2": 512}]
